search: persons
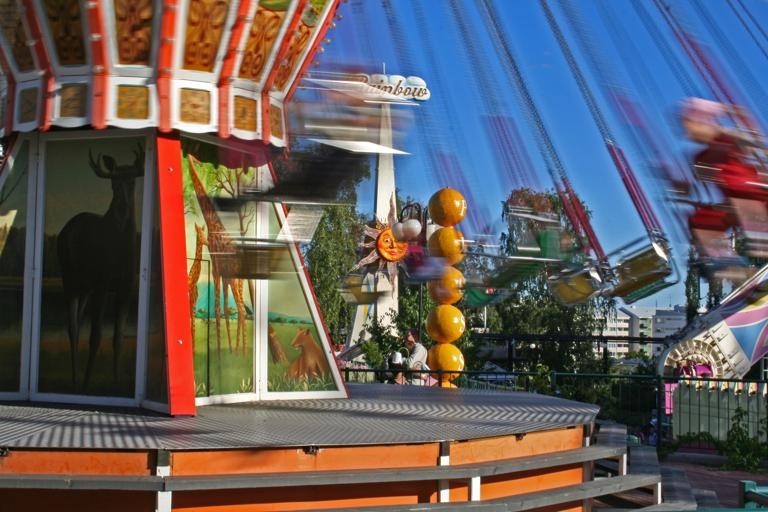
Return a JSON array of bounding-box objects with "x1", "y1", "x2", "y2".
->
[
  {"x1": 673, "y1": 95, "x2": 767, "y2": 230},
  {"x1": 388, "y1": 328, "x2": 428, "y2": 386}
]
